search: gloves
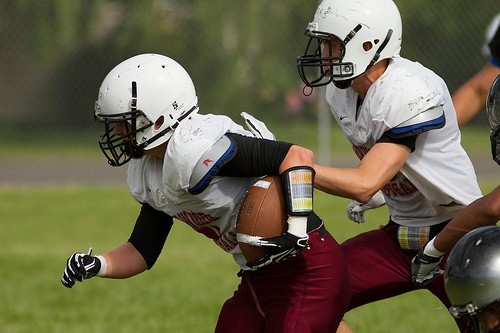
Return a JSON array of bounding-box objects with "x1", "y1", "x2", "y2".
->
[
  {"x1": 346, "y1": 189, "x2": 387, "y2": 224},
  {"x1": 240, "y1": 111, "x2": 276, "y2": 142},
  {"x1": 246, "y1": 232, "x2": 307, "y2": 272},
  {"x1": 61, "y1": 247, "x2": 102, "y2": 288},
  {"x1": 411, "y1": 236, "x2": 444, "y2": 287}
]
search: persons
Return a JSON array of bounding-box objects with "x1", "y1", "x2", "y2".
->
[
  {"x1": 444, "y1": 225, "x2": 500, "y2": 333},
  {"x1": 411, "y1": 74, "x2": 500, "y2": 283},
  {"x1": 451, "y1": 12, "x2": 500, "y2": 130},
  {"x1": 241, "y1": 0, "x2": 484, "y2": 333},
  {"x1": 60, "y1": 53, "x2": 348, "y2": 333}
]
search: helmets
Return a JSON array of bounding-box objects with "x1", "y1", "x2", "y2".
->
[
  {"x1": 307, "y1": 0, "x2": 402, "y2": 89},
  {"x1": 443, "y1": 226, "x2": 500, "y2": 333},
  {"x1": 486, "y1": 73, "x2": 500, "y2": 167},
  {"x1": 94, "y1": 53, "x2": 199, "y2": 159}
]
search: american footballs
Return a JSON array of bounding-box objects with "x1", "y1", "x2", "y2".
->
[{"x1": 235, "y1": 174, "x2": 288, "y2": 262}]
